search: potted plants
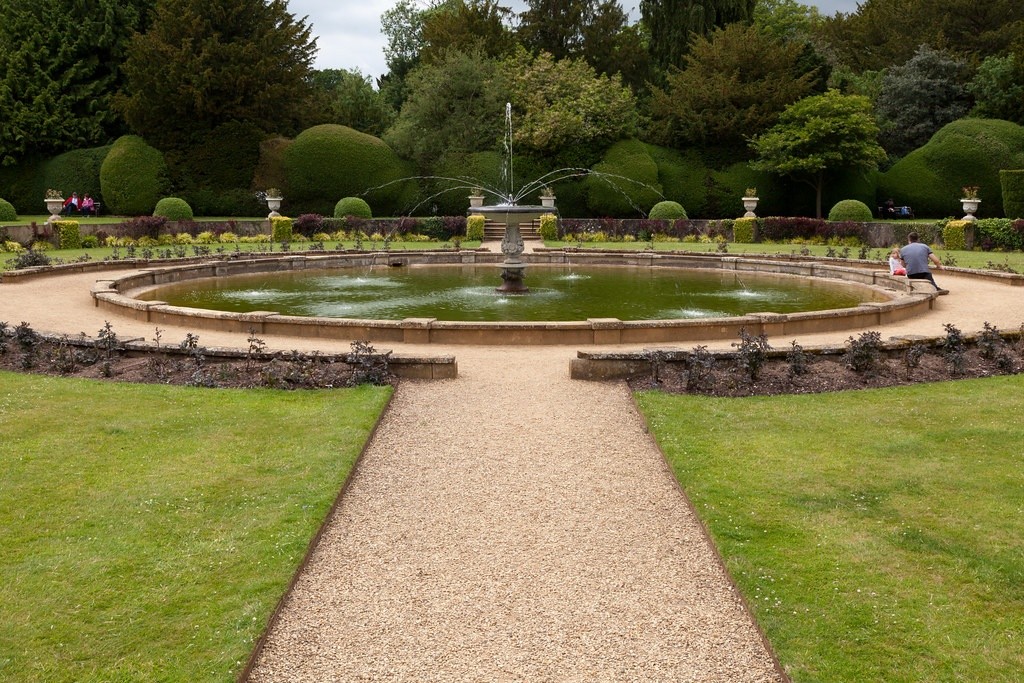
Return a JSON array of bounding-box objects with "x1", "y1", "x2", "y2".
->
[
  {"x1": 742, "y1": 188, "x2": 759, "y2": 211},
  {"x1": 43, "y1": 189, "x2": 65, "y2": 215},
  {"x1": 468, "y1": 187, "x2": 484, "y2": 207},
  {"x1": 539, "y1": 186, "x2": 556, "y2": 206},
  {"x1": 265, "y1": 188, "x2": 283, "y2": 210},
  {"x1": 959, "y1": 186, "x2": 981, "y2": 213}
]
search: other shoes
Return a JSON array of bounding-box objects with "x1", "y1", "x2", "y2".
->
[
  {"x1": 87, "y1": 215, "x2": 90, "y2": 218},
  {"x1": 937, "y1": 288, "x2": 949, "y2": 295},
  {"x1": 82, "y1": 216, "x2": 84, "y2": 218}
]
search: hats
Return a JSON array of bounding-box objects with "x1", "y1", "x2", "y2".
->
[{"x1": 73, "y1": 192, "x2": 77, "y2": 195}]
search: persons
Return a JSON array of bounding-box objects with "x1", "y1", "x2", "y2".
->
[
  {"x1": 882, "y1": 198, "x2": 897, "y2": 220},
  {"x1": 889, "y1": 248, "x2": 907, "y2": 276},
  {"x1": 899, "y1": 232, "x2": 947, "y2": 297},
  {"x1": 62, "y1": 192, "x2": 96, "y2": 218}
]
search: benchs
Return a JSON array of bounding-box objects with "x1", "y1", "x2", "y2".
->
[{"x1": 72, "y1": 202, "x2": 100, "y2": 218}]
